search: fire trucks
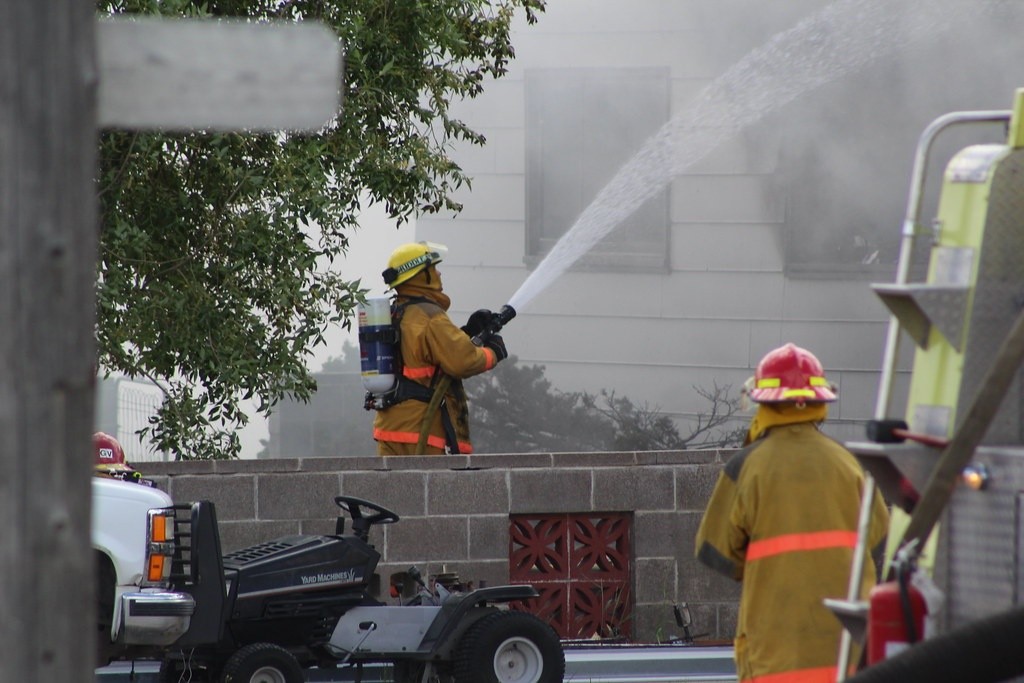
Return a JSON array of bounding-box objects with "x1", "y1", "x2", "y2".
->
[{"x1": 823, "y1": 84, "x2": 1024, "y2": 682}]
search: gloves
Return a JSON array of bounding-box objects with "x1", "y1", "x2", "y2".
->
[
  {"x1": 460, "y1": 309, "x2": 498, "y2": 339},
  {"x1": 479, "y1": 333, "x2": 507, "y2": 362}
]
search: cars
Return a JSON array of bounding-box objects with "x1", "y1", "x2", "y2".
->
[{"x1": 88, "y1": 478, "x2": 196, "y2": 665}]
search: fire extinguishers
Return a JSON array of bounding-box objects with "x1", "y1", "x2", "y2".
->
[{"x1": 867, "y1": 537, "x2": 928, "y2": 668}]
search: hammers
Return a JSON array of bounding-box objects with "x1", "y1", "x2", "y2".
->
[{"x1": 866, "y1": 419, "x2": 949, "y2": 448}]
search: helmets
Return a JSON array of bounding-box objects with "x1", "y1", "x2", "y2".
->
[
  {"x1": 741, "y1": 343, "x2": 839, "y2": 402},
  {"x1": 382, "y1": 241, "x2": 442, "y2": 294}
]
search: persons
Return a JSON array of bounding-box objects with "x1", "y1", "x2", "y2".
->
[
  {"x1": 372, "y1": 242, "x2": 508, "y2": 455},
  {"x1": 696, "y1": 345, "x2": 891, "y2": 683}
]
